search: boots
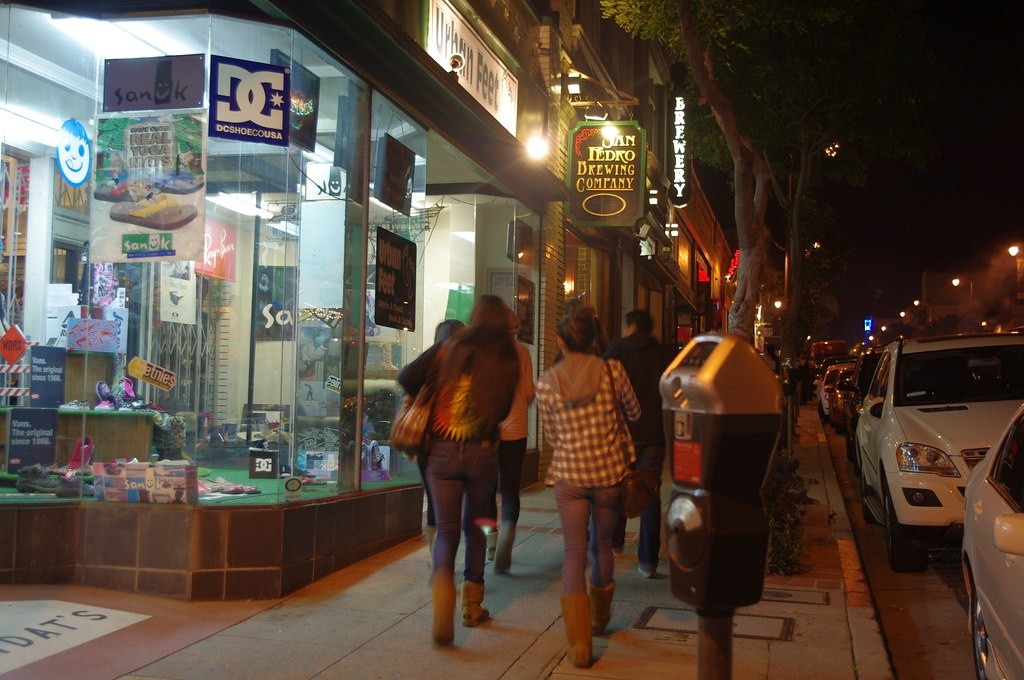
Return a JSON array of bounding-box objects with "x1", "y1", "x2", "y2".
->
[
  {"x1": 432, "y1": 568, "x2": 457, "y2": 645},
  {"x1": 589, "y1": 580, "x2": 616, "y2": 634},
  {"x1": 486, "y1": 531, "x2": 499, "y2": 560},
  {"x1": 460, "y1": 580, "x2": 490, "y2": 627},
  {"x1": 493, "y1": 518, "x2": 516, "y2": 573},
  {"x1": 559, "y1": 593, "x2": 594, "y2": 668},
  {"x1": 380, "y1": 343, "x2": 399, "y2": 370}
]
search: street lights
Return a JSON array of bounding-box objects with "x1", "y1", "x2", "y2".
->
[{"x1": 952, "y1": 275, "x2": 973, "y2": 302}]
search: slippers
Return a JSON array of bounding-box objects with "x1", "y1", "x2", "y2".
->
[
  {"x1": 150, "y1": 169, "x2": 204, "y2": 194},
  {"x1": 94, "y1": 175, "x2": 162, "y2": 203},
  {"x1": 110, "y1": 192, "x2": 198, "y2": 230}
]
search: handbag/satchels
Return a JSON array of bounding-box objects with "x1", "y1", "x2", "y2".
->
[
  {"x1": 617, "y1": 469, "x2": 659, "y2": 519},
  {"x1": 387, "y1": 381, "x2": 437, "y2": 450}
]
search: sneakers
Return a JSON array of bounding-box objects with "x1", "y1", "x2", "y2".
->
[
  {"x1": 362, "y1": 441, "x2": 369, "y2": 471},
  {"x1": 68, "y1": 437, "x2": 95, "y2": 470},
  {"x1": 56, "y1": 474, "x2": 95, "y2": 497},
  {"x1": 369, "y1": 440, "x2": 385, "y2": 471},
  {"x1": 15, "y1": 463, "x2": 57, "y2": 493}
]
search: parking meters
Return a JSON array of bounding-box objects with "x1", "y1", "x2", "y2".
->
[{"x1": 657, "y1": 335, "x2": 784, "y2": 680}]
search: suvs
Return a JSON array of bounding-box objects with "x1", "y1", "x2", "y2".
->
[{"x1": 853, "y1": 332, "x2": 1024, "y2": 571}]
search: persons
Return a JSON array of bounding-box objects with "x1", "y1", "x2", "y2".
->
[
  {"x1": 602, "y1": 310, "x2": 669, "y2": 579},
  {"x1": 551, "y1": 298, "x2": 606, "y2": 362},
  {"x1": 416, "y1": 321, "x2": 465, "y2": 570},
  {"x1": 536, "y1": 307, "x2": 642, "y2": 669},
  {"x1": 764, "y1": 344, "x2": 815, "y2": 403},
  {"x1": 400, "y1": 295, "x2": 519, "y2": 642},
  {"x1": 483, "y1": 307, "x2": 534, "y2": 577}
]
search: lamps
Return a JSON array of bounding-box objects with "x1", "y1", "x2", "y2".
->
[
  {"x1": 451, "y1": 53, "x2": 465, "y2": 71},
  {"x1": 585, "y1": 103, "x2": 609, "y2": 121},
  {"x1": 638, "y1": 224, "x2": 651, "y2": 239}
]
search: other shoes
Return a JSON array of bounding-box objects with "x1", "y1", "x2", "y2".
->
[
  {"x1": 197, "y1": 475, "x2": 261, "y2": 494},
  {"x1": 280, "y1": 464, "x2": 328, "y2": 485},
  {"x1": 612, "y1": 546, "x2": 624, "y2": 557},
  {"x1": 638, "y1": 561, "x2": 658, "y2": 578},
  {"x1": 248, "y1": 439, "x2": 269, "y2": 451},
  {"x1": 60, "y1": 380, "x2": 168, "y2": 413}
]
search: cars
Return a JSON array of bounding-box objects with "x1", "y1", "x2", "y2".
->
[
  {"x1": 820, "y1": 355, "x2": 882, "y2": 459},
  {"x1": 963, "y1": 406, "x2": 1024, "y2": 680}
]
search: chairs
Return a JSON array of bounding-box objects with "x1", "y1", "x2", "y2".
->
[{"x1": 908, "y1": 369, "x2": 973, "y2": 402}]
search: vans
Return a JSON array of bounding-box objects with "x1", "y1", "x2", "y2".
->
[{"x1": 814, "y1": 340, "x2": 849, "y2": 367}]
